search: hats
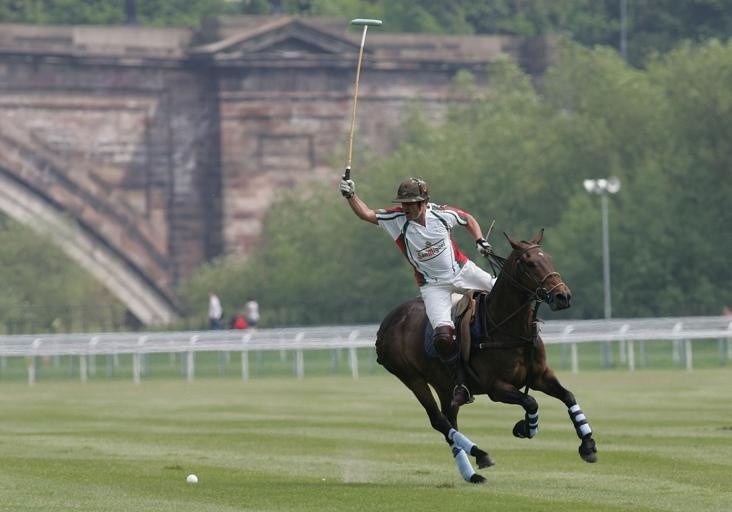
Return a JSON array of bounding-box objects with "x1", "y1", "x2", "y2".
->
[{"x1": 392, "y1": 177, "x2": 430, "y2": 203}]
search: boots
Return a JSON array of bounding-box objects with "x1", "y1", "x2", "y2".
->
[{"x1": 441, "y1": 354, "x2": 472, "y2": 402}]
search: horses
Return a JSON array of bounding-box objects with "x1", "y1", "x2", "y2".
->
[{"x1": 374, "y1": 227, "x2": 598, "y2": 484}]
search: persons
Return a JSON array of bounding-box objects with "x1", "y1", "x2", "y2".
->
[
  {"x1": 339, "y1": 173, "x2": 500, "y2": 407},
  {"x1": 230, "y1": 307, "x2": 250, "y2": 330},
  {"x1": 242, "y1": 294, "x2": 260, "y2": 328},
  {"x1": 206, "y1": 288, "x2": 226, "y2": 329}
]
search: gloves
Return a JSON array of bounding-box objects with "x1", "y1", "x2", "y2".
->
[
  {"x1": 340, "y1": 175, "x2": 355, "y2": 199},
  {"x1": 476, "y1": 238, "x2": 491, "y2": 255}
]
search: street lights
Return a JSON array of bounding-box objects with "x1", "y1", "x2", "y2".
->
[{"x1": 581, "y1": 175, "x2": 623, "y2": 369}]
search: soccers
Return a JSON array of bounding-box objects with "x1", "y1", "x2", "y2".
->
[{"x1": 185, "y1": 474, "x2": 200, "y2": 488}]
